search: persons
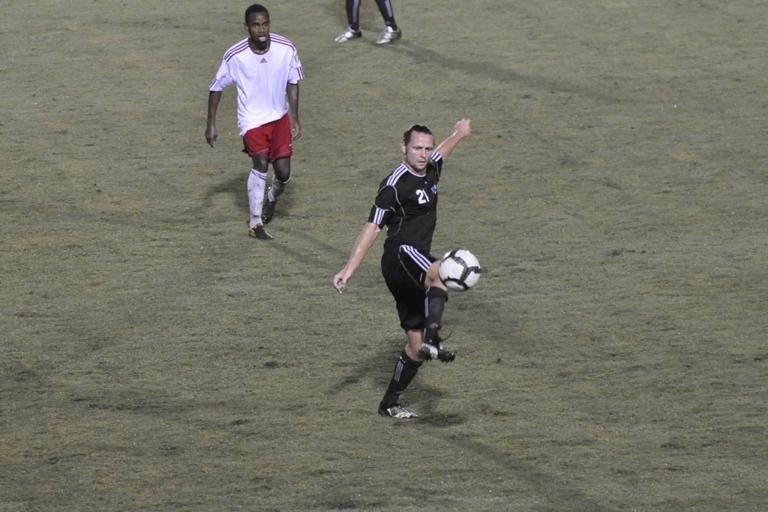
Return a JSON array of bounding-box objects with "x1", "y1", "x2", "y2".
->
[
  {"x1": 333, "y1": 1, "x2": 402, "y2": 45},
  {"x1": 331, "y1": 117, "x2": 473, "y2": 422},
  {"x1": 204, "y1": 3, "x2": 304, "y2": 239}
]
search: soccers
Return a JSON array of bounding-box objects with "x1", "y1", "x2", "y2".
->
[{"x1": 438, "y1": 251, "x2": 481, "y2": 292}]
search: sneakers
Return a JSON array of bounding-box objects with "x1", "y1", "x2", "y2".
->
[
  {"x1": 376, "y1": 25, "x2": 401, "y2": 45},
  {"x1": 246, "y1": 220, "x2": 275, "y2": 240},
  {"x1": 261, "y1": 194, "x2": 277, "y2": 223},
  {"x1": 334, "y1": 27, "x2": 362, "y2": 43},
  {"x1": 377, "y1": 400, "x2": 418, "y2": 420},
  {"x1": 416, "y1": 342, "x2": 457, "y2": 362}
]
